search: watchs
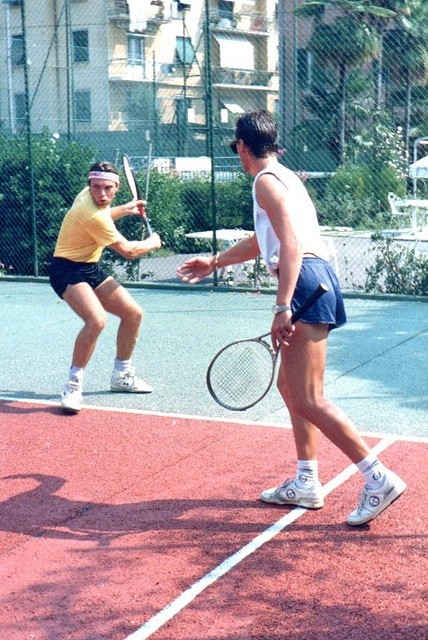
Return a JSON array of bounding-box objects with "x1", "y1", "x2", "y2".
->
[{"x1": 270, "y1": 301, "x2": 289, "y2": 315}]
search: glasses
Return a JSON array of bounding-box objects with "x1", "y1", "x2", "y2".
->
[{"x1": 229, "y1": 139, "x2": 240, "y2": 154}]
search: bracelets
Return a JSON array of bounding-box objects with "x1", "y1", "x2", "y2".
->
[{"x1": 213, "y1": 252, "x2": 219, "y2": 271}]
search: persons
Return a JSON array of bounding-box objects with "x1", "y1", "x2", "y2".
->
[
  {"x1": 176, "y1": 109, "x2": 408, "y2": 526},
  {"x1": 47, "y1": 163, "x2": 152, "y2": 414}
]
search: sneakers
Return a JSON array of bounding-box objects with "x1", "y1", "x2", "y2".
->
[
  {"x1": 261, "y1": 479, "x2": 325, "y2": 510},
  {"x1": 111, "y1": 376, "x2": 152, "y2": 393},
  {"x1": 61, "y1": 390, "x2": 82, "y2": 411},
  {"x1": 347, "y1": 470, "x2": 407, "y2": 526}
]
